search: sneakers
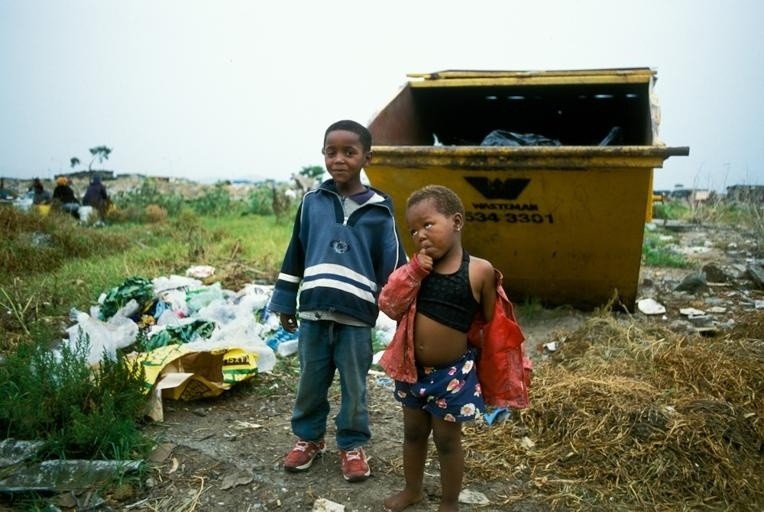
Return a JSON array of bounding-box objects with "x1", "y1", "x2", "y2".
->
[
  {"x1": 339, "y1": 446, "x2": 370, "y2": 481},
  {"x1": 284, "y1": 438, "x2": 327, "y2": 471}
]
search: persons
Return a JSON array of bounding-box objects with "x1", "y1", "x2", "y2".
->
[
  {"x1": 0, "y1": 176, "x2": 114, "y2": 227},
  {"x1": 267, "y1": 119, "x2": 410, "y2": 485},
  {"x1": 376, "y1": 183, "x2": 533, "y2": 511}
]
